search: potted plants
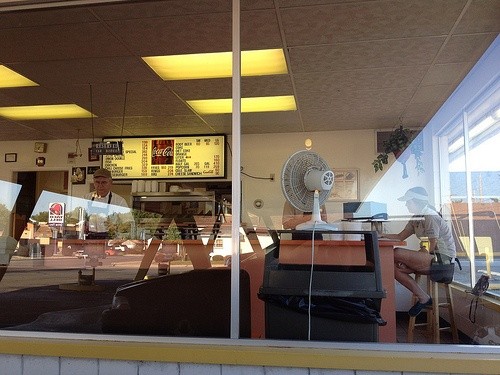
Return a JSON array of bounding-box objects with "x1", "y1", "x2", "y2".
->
[{"x1": 372, "y1": 120, "x2": 423, "y2": 178}]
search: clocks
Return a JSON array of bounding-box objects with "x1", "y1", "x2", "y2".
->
[{"x1": 34, "y1": 142, "x2": 46, "y2": 153}]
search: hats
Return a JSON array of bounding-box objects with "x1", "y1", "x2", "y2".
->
[
  {"x1": 93, "y1": 168, "x2": 110, "y2": 178},
  {"x1": 397, "y1": 190, "x2": 428, "y2": 203}
]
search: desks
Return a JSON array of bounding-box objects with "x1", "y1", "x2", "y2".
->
[{"x1": 28, "y1": 234, "x2": 408, "y2": 343}]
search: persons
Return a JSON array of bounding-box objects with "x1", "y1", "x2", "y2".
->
[
  {"x1": 77, "y1": 167, "x2": 136, "y2": 243},
  {"x1": 376, "y1": 186, "x2": 457, "y2": 318},
  {"x1": 282, "y1": 198, "x2": 328, "y2": 230}
]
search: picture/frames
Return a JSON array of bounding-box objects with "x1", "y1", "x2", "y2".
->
[{"x1": 5, "y1": 153, "x2": 17, "y2": 162}]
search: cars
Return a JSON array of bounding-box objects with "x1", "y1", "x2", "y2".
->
[{"x1": 71, "y1": 244, "x2": 179, "y2": 265}]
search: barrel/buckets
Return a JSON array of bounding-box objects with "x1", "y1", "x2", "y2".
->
[{"x1": 343, "y1": 222, "x2": 362, "y2": 241}]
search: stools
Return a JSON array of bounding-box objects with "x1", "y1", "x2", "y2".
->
[{"x1": 407, "y1": 270, "x2": 460, "y2": 347}]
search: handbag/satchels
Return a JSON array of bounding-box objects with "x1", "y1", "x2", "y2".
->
[
  {"x1": 471, "y1": 275, "x2": 490, "y2": 295},
  {"x1": 430, "y1": 258, "x2": 454, "y2": 283}
]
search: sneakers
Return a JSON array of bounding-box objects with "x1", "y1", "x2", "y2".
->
[{"x1": 408, "y1": 297, "x2": 432, "y2": 317}]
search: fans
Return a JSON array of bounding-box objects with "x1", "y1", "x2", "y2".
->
[{"x1": 281, "y1": 150, "x2": 339, "y2": 232}]
